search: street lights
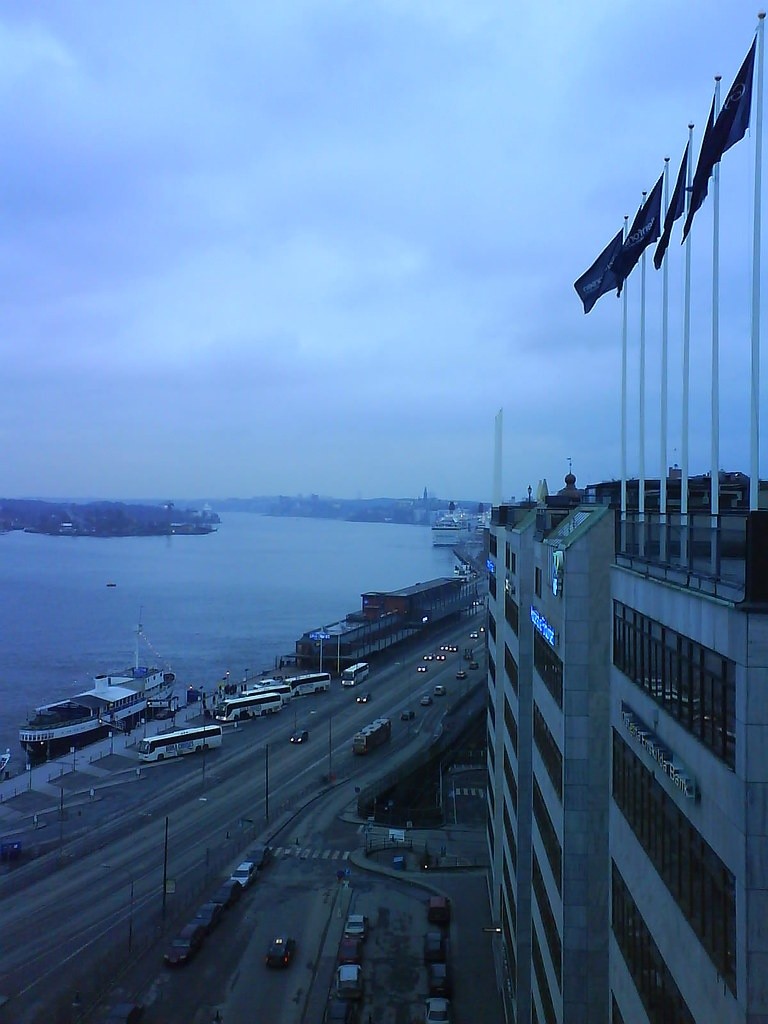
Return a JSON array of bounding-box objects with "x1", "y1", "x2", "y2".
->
[{"x1": 225, "y1": 671, "x2": 230, "y2": 684}]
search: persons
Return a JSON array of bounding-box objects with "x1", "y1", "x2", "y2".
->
[
  {"x1": 279, "y1": 664, "x2": 281, "y2": 671},
  {"x1": 124, "y1": 725, "x2": 132, "y2": 736},
  {"x1": 273, "y1": 674, "x2": 293, "y2": 681},
  {"x1": 212, "y1": 685, "x2": 248, "y2": 704},
  {"x1": 203, "y1": 700, "x2": 207, "y2": 709},
  {"x1": 233, "y1": 715, "x2": 240, "y2": 726},
  {"x1": 252, "y1": 712, "x2": 256, "y2": 719}
]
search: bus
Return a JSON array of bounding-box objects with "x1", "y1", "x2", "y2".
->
[
  {"x1": 137, "y1": 724, "x2": 223, "y2": 762},
  {"x1": 352, "y1": 718, "x2": 392, "y2": 754},
  {"x1": 341, "y1": 662, "x2": 369, "y2": 686},
  {"x1": 215, "y1": 692, "x2": 283, "y2": 721},
  {"x1": 282, "y1": 673, "x2": 332, "y2": 697},
  {"x1": 241, "y1": 685, "x2": 293, "y2": 706}
]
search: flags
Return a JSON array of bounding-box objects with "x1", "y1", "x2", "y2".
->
[
  {"x1": 611, "y1": 172, "x2": 666, "y2": 298},
  {"x1": 653, "y1": 140, "x2": 689, "y2": 271},
  {"x1": 573, "y1": 227, "x2": 624, "y2": 315},
  {"x1": 681, "y1": 32, "x2": 757, "y2": 246}
]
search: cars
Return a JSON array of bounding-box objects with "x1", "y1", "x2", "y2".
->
[
  {"x1": 289, "y1": 731, "x2": 308, "y2": 744},
  {"x1": 268, "y1": 936, "x2": 295, "y2": 967},
  {"x1": 401, "y1": 710, "x2": 415, "y2": 720},
  {"x1": 415, "y1": 623, "x2": 486, "y2": 707},
  {"x1": 320, "y1": 895, "x2": 453, "y2": 1024},
  {"x1": 356, "y1": 692, "x2": 371, "y2": 703},
  {"x1": 162, "y1": 844, "x2": 275, "y2": 966}
]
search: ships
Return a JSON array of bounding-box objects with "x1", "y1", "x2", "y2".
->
[
  {"x1": 429, "y1": 513, "x2": 485, "y2": 547},
  {"x1": 18, "y1": 666, "x2": 177, "y2": 762}
]
